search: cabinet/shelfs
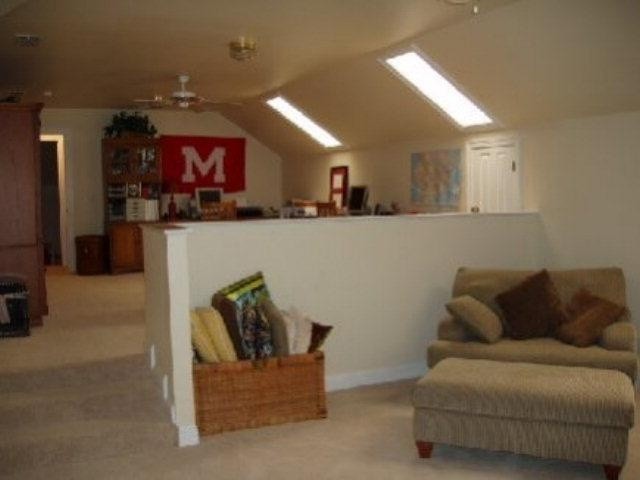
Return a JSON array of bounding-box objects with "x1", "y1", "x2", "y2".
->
[
  {"x1": 101, "y1": 135, "x2": 163, "y2": 277},
  {"x1": 0, "y1": 100, "x2": 47, "y2": 330}
]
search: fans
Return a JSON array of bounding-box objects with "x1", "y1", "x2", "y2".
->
[{"x1": 129, "y1": 73, "x2": 248, "y2": 112}]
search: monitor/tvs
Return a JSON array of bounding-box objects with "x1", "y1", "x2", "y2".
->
[
  {"x1": 194, "y1": 188, "x2": 224, "y2": 213},
  {"x1": 346, "y1": 185, "x2": 371, "y2": 214}
]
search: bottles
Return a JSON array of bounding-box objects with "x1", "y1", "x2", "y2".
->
[{"x1": 167, "y1": 194, "x2": 177, "y2": 222}]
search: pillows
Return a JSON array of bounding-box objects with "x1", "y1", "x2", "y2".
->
[
  {"x1": 492, "y1": 267, "x2": 567, "y2": 342},
  {"x1": 559, "y1": 287, "x2": 625, "y2": 346},
  {"x1": 442, "y1": 293, "x2": 504, "y2": 345}
]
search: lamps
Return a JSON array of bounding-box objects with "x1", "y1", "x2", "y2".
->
[{"x1": 227, "y1": 37, "x2": 262, "y2": 68}]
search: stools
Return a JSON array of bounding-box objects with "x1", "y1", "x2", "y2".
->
[{"x1": 410, "y1": 352, "x2": 640, "y2": 480}]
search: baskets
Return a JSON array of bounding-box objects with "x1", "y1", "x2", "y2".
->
[{"x1": 195, "y1": 350, "x2": 329, "y2": 435}]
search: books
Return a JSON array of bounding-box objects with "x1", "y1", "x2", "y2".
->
[{"x1": 109, "y1": 183, "x2": 161, "y2": 222}]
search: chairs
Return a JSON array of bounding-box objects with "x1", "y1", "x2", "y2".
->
[
  {"x1": 424, "y1": 264, "x2": 640, "y2": 394},
  {"x1": 315, "y1": 200, "x2": 338, "y2": 217},
  {"x1": 199, "y1": 198, "x2": 238, "y2": 221}
]
